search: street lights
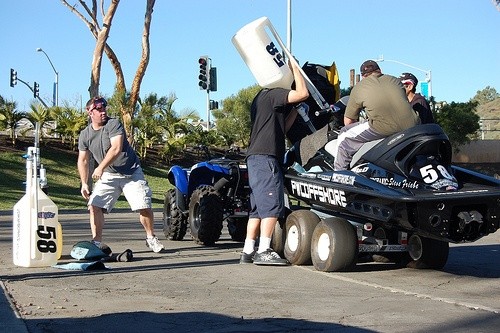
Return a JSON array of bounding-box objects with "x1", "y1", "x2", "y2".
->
[
  {"x1": 374, "y1": 59, "x2": 433, "y2": 114},
  {"x1": 36, "y1": 48, "x2": 59, "y2": 138}
]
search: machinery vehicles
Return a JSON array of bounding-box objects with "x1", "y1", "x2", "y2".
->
[{"x1": 162, "y1": 156, "x2": 250, "y2": 247}]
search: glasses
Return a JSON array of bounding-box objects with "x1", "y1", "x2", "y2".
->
[{"x1": 94, "y1": 98, "x2": 108, "y2": 110}]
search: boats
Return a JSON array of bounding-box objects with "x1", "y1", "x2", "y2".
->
[{"x1": 281, "y1": 163, "x2": 500, "y2": 245}]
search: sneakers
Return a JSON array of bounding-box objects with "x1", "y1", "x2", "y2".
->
[
  {"x1": 146, "y1": 236, "x2": 165, "y2": 252},
  {"x1": 92, "y1": 240, "x2": 101, "y2": 246},
  {"x1": 239, "y1": 250, "x2": 256, "y2": 264},
  {"x1": 253, "y1": 248, "x2": 289, "y2": 265}
]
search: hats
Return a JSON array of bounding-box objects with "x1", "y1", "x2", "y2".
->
[
  {"x1": 398, "y1": 73, "x2": 418, "y2": 86},
  {"x1": 360, "y1": 60, "x2": 379, "y2": 74}
]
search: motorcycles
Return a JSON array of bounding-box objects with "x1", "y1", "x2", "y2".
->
[{"x1": 313, "y1": 94, "x2": 460, "y2": 193}]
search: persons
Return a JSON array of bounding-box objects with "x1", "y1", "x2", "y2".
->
[
  {"x1": 335, "y1": 60, "x2": 421, "y2": 171},
  {"x1": 240, "y1": 56, "x2": 309, "y2": 265},
  {"x1": 397, "y1": 72, "x2": 433, "y2": 124},
  {"x1": 77, "y1": 97, "x2": 164, "y2": 253}
]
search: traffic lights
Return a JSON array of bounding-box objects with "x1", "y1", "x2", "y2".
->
[
  {"x1": 198, "y1": 55, "x2": 209, "y2": 90},
  {"x1": 10, "y1": 68, "x2": 17, "y2": 87},
  {"x1": 34, "y1": 81, "x2": 39, "y2": 98}
]
